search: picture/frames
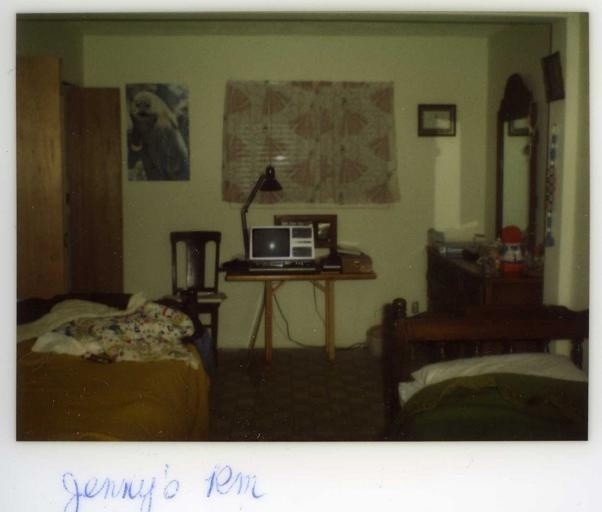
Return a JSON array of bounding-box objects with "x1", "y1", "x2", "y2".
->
[{"x1": 417, "y1": 104, "x2": 456, "y2": 136}]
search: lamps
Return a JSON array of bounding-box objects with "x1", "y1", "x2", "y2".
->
[{"x1": 235, "y1": 166, "x2": 283, "y2": 273}]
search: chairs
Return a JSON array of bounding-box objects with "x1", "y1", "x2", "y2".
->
[{"x1": 168, "y1": 231, "x2": 228, "y2": 375}]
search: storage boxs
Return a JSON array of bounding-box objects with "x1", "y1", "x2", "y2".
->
[{"x1": 339, "y1": 254, "x2": 372, "y2": 274}]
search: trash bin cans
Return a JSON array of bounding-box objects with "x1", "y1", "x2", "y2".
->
[{"x1": 367, "y1": 327, "x2": 384, "y2": 358}]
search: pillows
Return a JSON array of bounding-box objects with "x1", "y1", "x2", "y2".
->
[{"x1": 411, "y1": 352, "x2": 588, "y2": 388}]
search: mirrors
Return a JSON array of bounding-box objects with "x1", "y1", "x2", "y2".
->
[{"x1": 496, "y1": 73, "x2": 538, "y2": 249}]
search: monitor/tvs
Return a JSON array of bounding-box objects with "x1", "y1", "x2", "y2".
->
[{"x1": 249, "y1": 226, "x2": 315, "y2": 260}]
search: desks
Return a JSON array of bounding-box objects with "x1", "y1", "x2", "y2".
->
[{"x1": 225, "y1": 270, "x2": 378, "y2": 364}]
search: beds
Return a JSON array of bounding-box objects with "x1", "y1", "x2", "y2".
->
[
  {"x1": 388, "y1": 298, "x2": 589, "y2": 441},
  {"x1": 17, "y1": 294, "x2": 209, "y2": 441}
]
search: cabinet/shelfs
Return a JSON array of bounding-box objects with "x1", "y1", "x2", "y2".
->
[
  {"x1": 429, "y1": 246, "x2": 544, "y2": 318},
  {"x1": 20, "y1": 56, "x2": 126, "y2": 305}
]
